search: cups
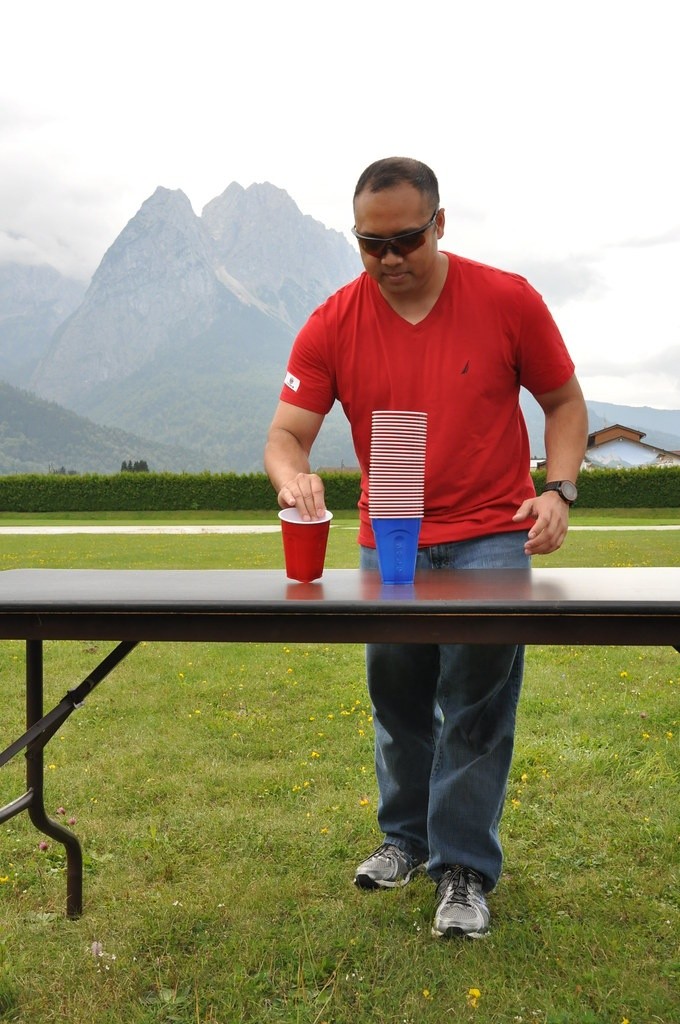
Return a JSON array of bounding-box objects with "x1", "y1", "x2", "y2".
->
[
  {"x1": 367, "y1": 410, "x2": 428, "y2": 584},
  {"x1": 278, "y1": 508, "x2": 333, "y2": 583}
]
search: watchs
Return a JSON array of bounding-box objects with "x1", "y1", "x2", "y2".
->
[{"x1": 542, "y1": 480, "x2": 578, "y2": 507}]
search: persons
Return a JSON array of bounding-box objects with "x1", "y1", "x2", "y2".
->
[{"x1": 264, "y1": 156, "x2": 588, "y2": 939}]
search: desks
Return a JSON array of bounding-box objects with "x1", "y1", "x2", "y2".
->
[{"x1": 0, "y1": 568, "x2": 680, "y2": 921}]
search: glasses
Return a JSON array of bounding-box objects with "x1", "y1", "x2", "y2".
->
[{"x1": 351, "y1": 208, "x2": 439, "y2": 259}]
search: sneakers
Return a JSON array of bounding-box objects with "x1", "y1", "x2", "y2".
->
[
  {"x1": 431, "y1": 864, "x2": 491, "y2": 942},
  {"x1": 354, "y1": 843, "x2": 429, "y2": 892}
]
